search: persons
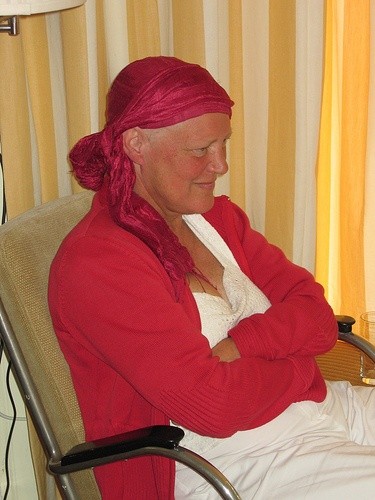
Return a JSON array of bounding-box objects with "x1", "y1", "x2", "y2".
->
[{"x1": 48, "y1": 57, "x2": 375, "y2": 500}]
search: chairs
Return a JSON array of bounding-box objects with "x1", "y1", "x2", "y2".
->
[{"x1": 0, "y1": 190, "x2": 375, "y2": 500}]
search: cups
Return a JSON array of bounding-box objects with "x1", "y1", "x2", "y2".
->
[{"x1": 359, "y1": 312, "x2": 375, "y2": 386}]
